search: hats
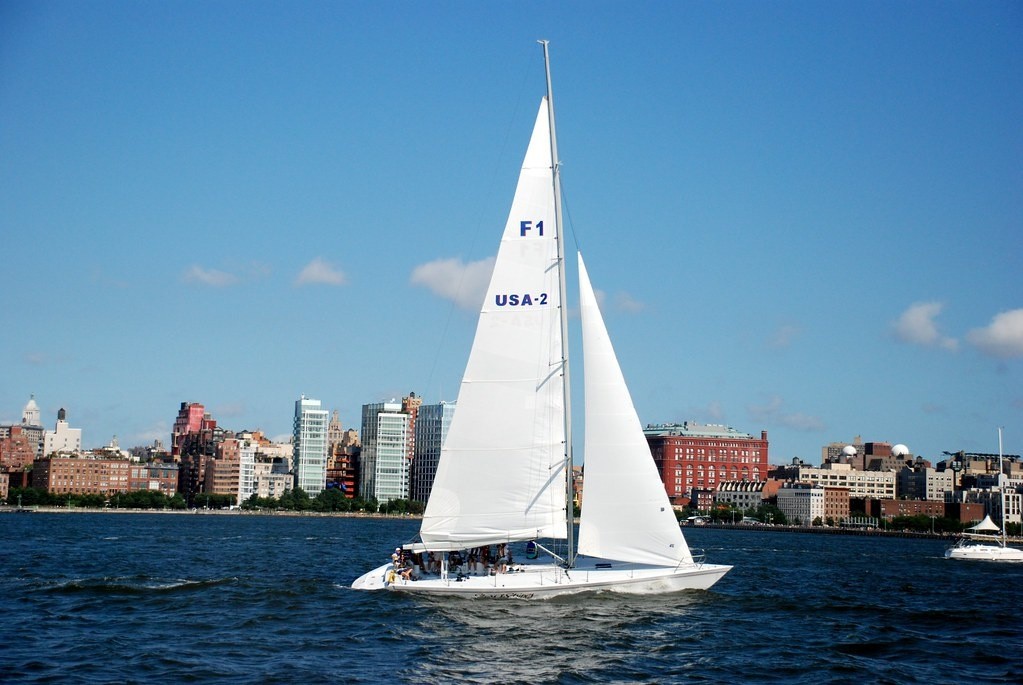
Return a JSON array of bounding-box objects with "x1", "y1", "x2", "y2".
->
[{"x1": 396, "y1": 548, "x2": 401, "y2": 550}]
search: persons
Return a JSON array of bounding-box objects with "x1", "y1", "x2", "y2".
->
[{"x1": 391, "y1": 543, "x2": 513, "y2": 580}]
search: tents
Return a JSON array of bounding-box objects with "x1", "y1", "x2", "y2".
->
[{"x1": 966, "y1": 515, "x2": 1001, "y2": 540}]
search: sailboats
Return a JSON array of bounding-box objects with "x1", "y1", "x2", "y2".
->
[
  {"x1": 352, "y1": 39, "x2": 735, "y2": 598},
  {"x1": 944, "y1": 423, "x2": 1023, "y2": 563}
]
